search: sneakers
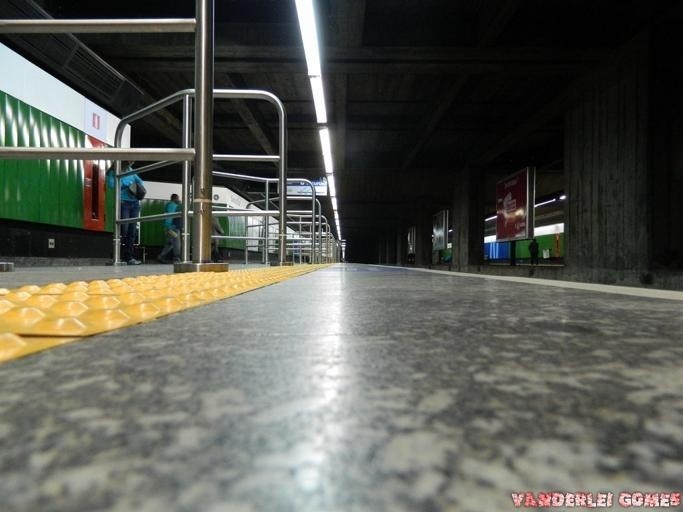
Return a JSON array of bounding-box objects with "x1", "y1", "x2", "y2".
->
[
  {"x1": 157, "y1": 255, "x2": 169, "y2": 264},
  {"x1": 105, "y1": 259, "x2": 114, "y2": 265},
  {"x1": 214, "y1": 259, "x2": 223, "y2": 263},
  {"x1": 129, "y1": 258, "x2": 142, "y2": 265}
]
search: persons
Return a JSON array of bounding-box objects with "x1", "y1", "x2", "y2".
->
[
  {"x1": 210, "y1": 217, "x2": 224, "y2": 258},
  {"x1": 157, "y1": 194, "x2": 184, "y2": 264},
  {"x1": 105, "y1": 158, "x2": 144, "y2": 265},
  {"x1": 528, "y1": 238, "x2": 539, "y2": 265}
]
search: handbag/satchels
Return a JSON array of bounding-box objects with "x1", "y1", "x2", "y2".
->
[{"x1": 127, "y1": 183, "x2": 147, "y2": 201}]
